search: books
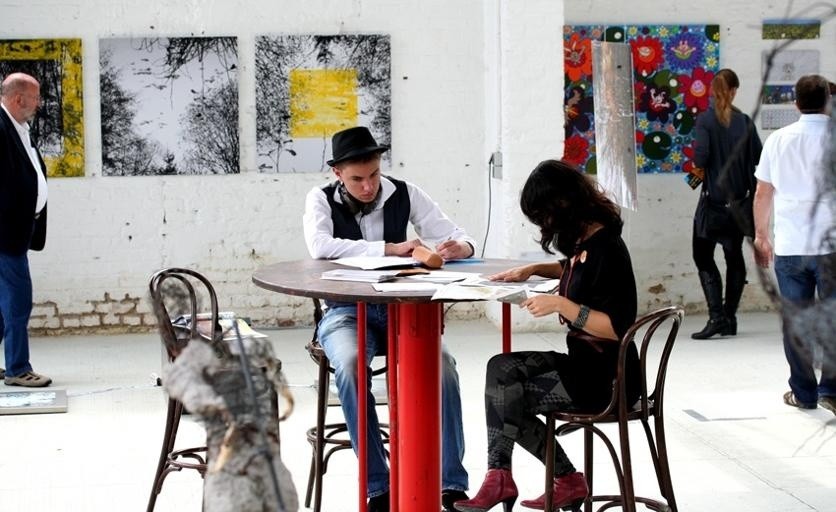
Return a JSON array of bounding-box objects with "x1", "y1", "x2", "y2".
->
[
  {"x1": 171, "y1": 312, "x2": 274, "y2": 357},
  {"x1": 0, "y1": 391, "x2": 69, "y2": 416}
]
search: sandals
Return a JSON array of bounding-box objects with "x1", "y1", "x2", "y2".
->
[
  {"x1": 783, "y1": 391, "x2": 814, "y2": 409},
  {"x1": 819, "y1": 396, "x2": 836, "y2": 415}
]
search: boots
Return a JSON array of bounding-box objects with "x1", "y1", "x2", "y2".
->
[
  {"x1": 691, "y1": 269, "x2": 728, "y2": 339},
  {"x1": 724, "y1": 272, "x2": 748, "y2": 335}
]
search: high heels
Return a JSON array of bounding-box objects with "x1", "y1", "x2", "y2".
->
[
  {"x1": 454, "y1": 469, "x2": 518, "y2": 512},
  {"x1": 520, "y1": 472, "x2": 589, "y2": 512}
]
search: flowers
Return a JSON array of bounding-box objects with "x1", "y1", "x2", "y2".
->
[
  {"x1": 677, "y1": 68, "x2": 717, "y2": 111},
  {"x1": 564, "y1": 34, "x2": 596, "y2": 82},
  {"x1": 628, "y1": 35, "x2": 665, "y2": 75},
  {"x1": 640, "y1": 82, "x2": 677, "y2": 123},
  {"x1": 566, "y1": 134, "x2": 590, "y2": 166},
  {"x1": 666, "y1": 34, "x2": 706, "y2": 70}
]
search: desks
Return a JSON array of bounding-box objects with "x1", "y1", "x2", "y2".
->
[{"x1": 253, "y1": 257, "x2": 562, "y2": 512}]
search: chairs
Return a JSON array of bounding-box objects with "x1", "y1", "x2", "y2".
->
[
  {"x1": 305, "y1": 296, "x2": 393, "y2": 512},
  {"x1": 544, "y1": 303, "x2": 686, "y2": 512},
  {"x1": 146, "y1": 265, "x2": 261, "y2": 512}
]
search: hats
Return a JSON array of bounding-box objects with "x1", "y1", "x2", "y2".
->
[{"x1": 327, "y1": 125, "x2": 389, "y2": 167}]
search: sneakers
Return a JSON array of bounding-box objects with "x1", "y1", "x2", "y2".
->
[
  {"x1": 367, "y1": 491, "x2": 390, "y2": 512},
  {"x1": 442, "y1": 489, "x2": 470, "y2": 512},
  {"x1": 4, "y1": 371, "x2": 52, "y2": 387},
  {"x1": 0, "y1": 366, "x2": 7, "y2": 378}
]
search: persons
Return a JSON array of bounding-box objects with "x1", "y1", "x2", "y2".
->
[
  {"x1": 0, "y1": 73, "x2": 52, "y2": 387},
  {"x1": 753, "y1": 74, "x2": 836, "y2": 415},
  {"x1": 691, "y1": 69, "x2": 763, "y2": 339},
  {"x1": 303, "y1": 125, "x2": 478, "y2": 512},
  {"x1": 452, "y1": 160, "x2": 637, "y2": 512}
]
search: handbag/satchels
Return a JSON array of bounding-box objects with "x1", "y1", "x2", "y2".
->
[{"x1": 718, "y1": 113, "x2": 758, "y2": 234}]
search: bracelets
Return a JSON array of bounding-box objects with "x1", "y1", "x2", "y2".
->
[{"x1": 574, "y1": 306, "x2": 591, "y2": 330}]
